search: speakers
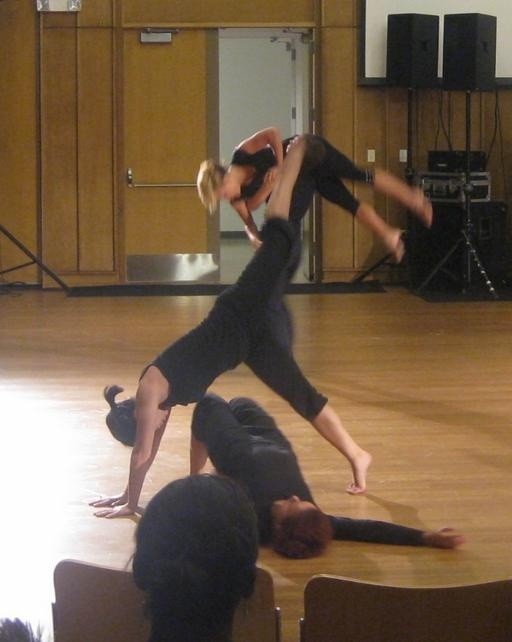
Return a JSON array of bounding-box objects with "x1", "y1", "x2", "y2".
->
[
  {"x1": 443, "y1": 14, "x2": 497, "y2": 89},
  {"x1": 387, "y1": 13, "x2": 438, "y2": 89}
]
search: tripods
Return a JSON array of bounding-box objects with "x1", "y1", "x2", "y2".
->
[
  {"x1": 351, "y1": 90, "x2": 417, "y2": 291},
  {"x1": 414, "y1": 90, "x2": 501, "y2": 300}
]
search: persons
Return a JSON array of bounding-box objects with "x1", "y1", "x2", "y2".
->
[
  {"x1": 189, "y1": 395, "x2": 464, "y2": 558},
  {"x1": 132, "y1": 473, "x2": 260, "y2": 642},
  {"x1": 197, "y1": 127, "x2": 434, "y2": 264},
  {"x1": 88, "y1": 137, "x2": 373, "y2": 518}
]
